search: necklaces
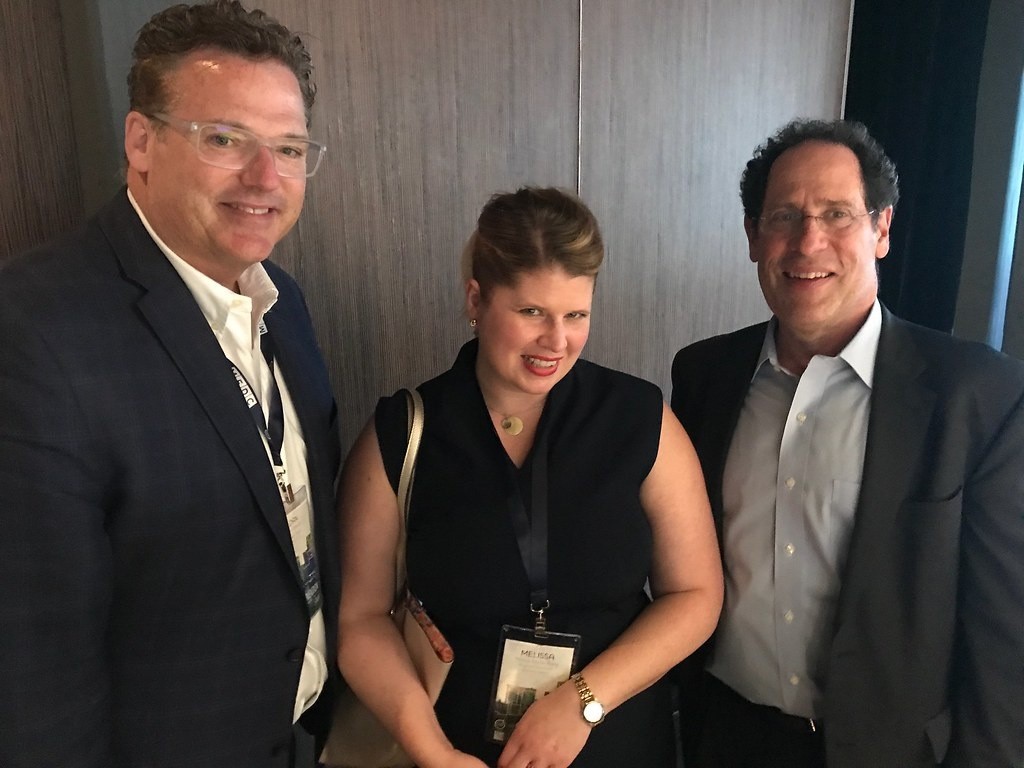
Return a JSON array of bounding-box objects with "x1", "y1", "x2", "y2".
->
[{"x1": 487, "y1": 403, "x2": 542, "y2": 436}]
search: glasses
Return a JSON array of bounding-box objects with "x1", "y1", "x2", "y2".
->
[
  {"x1": 152, "y1": 111, "x2": 328, "y2": 178},
  {"x1": 758, "y1": 207, "x2": 875, "y2": 231}
]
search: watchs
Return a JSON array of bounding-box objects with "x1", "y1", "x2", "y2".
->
[{"x1": 572, "y1": 672, "x2": 605, "y2": 727}]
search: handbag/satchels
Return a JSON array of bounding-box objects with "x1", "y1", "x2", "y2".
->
[{"x1": 318, "y1": 588, "x2": 455, "y2": 768}]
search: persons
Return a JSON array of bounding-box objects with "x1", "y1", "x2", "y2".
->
[
  {"x1": 0, "y1": 0, "x2": 339, "y2": 768},
  {"x1": 671, "y1": 115, "x2": 1024, "y2": 768},
  {"x1": 333, "y1": 186, "x2": 724, "y2": 768}
]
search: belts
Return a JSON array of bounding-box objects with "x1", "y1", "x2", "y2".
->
[{"x1": 701, "y1": 671, "x2": 825, "y2": 735}]
